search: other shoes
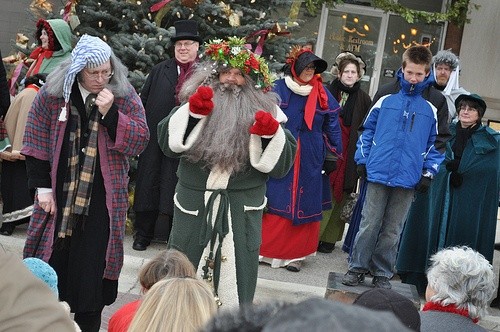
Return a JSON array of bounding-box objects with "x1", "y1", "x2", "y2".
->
[
  {"x1": 319, "y1": 241, "x2": 336, "y2": 253},
  {"x1": 133, "y1": 236, "x2": 151, "y2": 250},
  {"x1": 286, "y1": 260, "x2": 304, "y2": 272},
  {"x1": 0, "y1": 222, "x2": 16, "y2": 235},
  {"x1": 370, "y1": 276, "x2": 393, "y2": 289},
  {"x1": 340, "y1": 270, "x2": 365, "y2": 286}
]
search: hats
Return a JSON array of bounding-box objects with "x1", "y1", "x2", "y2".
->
[
  {"x1": 57, "y1": 33, "x2": 113, "y2": 122},
  {"x1": 353, "y1": 288, "x2": 421, "y2": 332},
  {"x1": 455, "y1": 93, "x2": 487, "y2": 117},
  {"x1": 171, "y1": 20, "x2": 204, "y2": 50}
]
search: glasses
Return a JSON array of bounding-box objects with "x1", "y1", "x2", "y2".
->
[
  {"x1": 174, "y1": 41, "x2": 196, "y2": 47},
  {"x1": 459, "y1": 107, "x2": 478, "y2": 112},
  {"x1": 80, "y1": 64, "x2": 116, "y2": 80},
  {"x1": 304, "y1": 65, "x2": 317, "y2": 70}
]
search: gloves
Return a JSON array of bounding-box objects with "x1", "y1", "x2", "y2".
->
[
  {"x1": 324, "y1": 161, "x2": 337, "y2": 175},
  {"x1": 356, "y1": 165, "x2": 368, "y2": 185},
  {"x1": 415, "y1": 176, "x2": 432, "y2": 192},
  {"x1": 450, "y1": 172, "x2": 464, "y2": 187},
  {"x1": 189, "y1": 87, "x2": 214, "y2": 119},
  {"x1": 248, "y1": 110, "x2": 279, "y2": 140},
  {"x1": 446, "y1": 160, "x2": 459, "y2": 172}
]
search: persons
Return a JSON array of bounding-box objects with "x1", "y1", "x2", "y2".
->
[
  {"x1": 199, "y1": 299, "x2": 301, "y2": 332},
  {"x1": 342, "y1": 47, "x2": 441, "y2": 289},
  {"x1": 0, "y1": 237, "x2": 77, "y2": 332},
  {"x1": 317, "y1": 51, "x2": 373, "y2": 253},
  {"x1": 21, "y1": 257, "x2": 82, "y2": 332},
  {"x1": 133, "y1": 20, "x2": 203, "y2": 251},
  {"x1": 353, "y1": 245, "x2": 500, "y2": 332},
  {"x1": 432, "y1": 47, "x2": 471, "y2": 124},
  {"x1": 262, "y1": 296, "x2": 413, "y2": 332},
  {"x1": 259, "y1": 45, "x2": 343, "y2": 273},
  {"x1": 0, "y1": 19, "x2": 73, "y2": 236},
  {"x1": 158, "y1": 36, "x2": 298, "y2": 315},
  {"x1": 21, "y1": 33, "x2": 150, "y2": 332},
  {"x1": 394, "y1": 93, "x2": 500, "y2": 299},
  {"x1": 126, "y1": 276, "x2": 219, "y2": 332},
  {"x1": 108, "y1": 248, "x2": 196, "y2": 332}
]
